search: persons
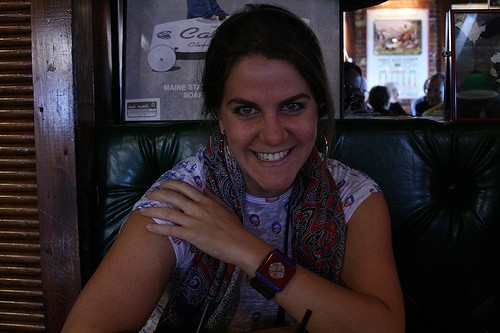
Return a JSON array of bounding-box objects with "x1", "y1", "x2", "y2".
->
[
  {"x1": 343, "y1": 62, "x2": 406, "y2": 116},
  {"x1": 411, "y1": 74, "x2": 446, "y2": 116},
  {"x1": 60, "y1": 2, "x2": 406, "y2": 333}
]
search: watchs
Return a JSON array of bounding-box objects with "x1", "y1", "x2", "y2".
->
[{"x1": 250, "y1": 248, "x2": 296, "y2": 300}]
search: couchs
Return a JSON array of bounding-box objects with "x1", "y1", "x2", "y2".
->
[{"x1": 94, "y1": 118, "x2": 500, "y2": 333}]
